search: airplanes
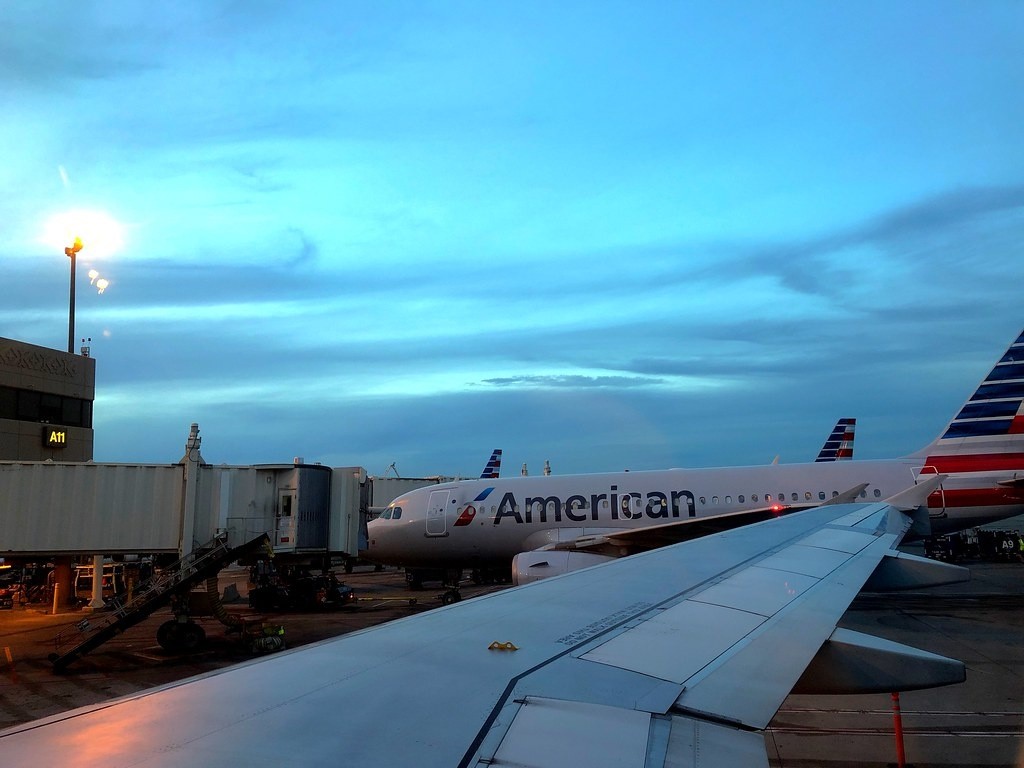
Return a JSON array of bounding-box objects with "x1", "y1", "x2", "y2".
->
[
  {"x1": 1, "y1": 505, "x2": 979, "y2": 767},
  {"x1": 361, "y1": 321, "x2": 1023, "y2": 591}
]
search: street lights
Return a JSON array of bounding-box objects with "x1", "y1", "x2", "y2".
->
[{"x1": 65, "y1": 241, "x2": 86, "y2": 353}]
